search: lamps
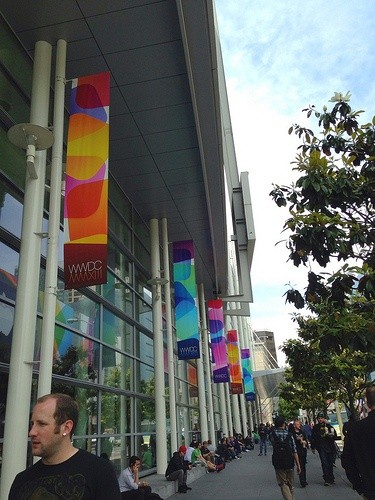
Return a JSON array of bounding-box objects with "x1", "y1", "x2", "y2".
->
[
  {"x1": 7, "y1": 123, "x2": 54, "y2": 178},
  {"x1": 148, "y1": 278, "x2": 169, "y2": 300}
]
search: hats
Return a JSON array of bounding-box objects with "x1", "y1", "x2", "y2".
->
[
  {"x1": 319, "y1": 413, "x2": 325, "y2": 417},
  {"x1": 179, "y1": 446, "x2": 186, "y2": 452}
]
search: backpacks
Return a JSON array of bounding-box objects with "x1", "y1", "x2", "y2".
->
[{"x1": 272, "y1": 430, "x2": 294, "y2": 469}]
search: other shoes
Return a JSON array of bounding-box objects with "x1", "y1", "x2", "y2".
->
[
  {"x1": 330, "y1": 481, "x2": 336, "y2": 484},
  {"x1": 302, "y1": 483, "x2": 306, "y2": 488},
  {"x1": 178, "y1": 489, "x2": 186, "y2": 493},
  {"x1": 324, "y1": 483, "x2": 331, "y2": 486},
  {"x1": 300, "y1": 482, "x2": 308, "y2": 485},
  {"x1": 217, "y1": 464, "x2": 225, "y2": 472},
  {"x1": 184, "y1": 486, "x2": 192, "y2": 490}
]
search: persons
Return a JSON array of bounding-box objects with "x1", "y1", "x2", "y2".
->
[
  {"x1": 310, "y1": 412, "x2": 338, "y2": 485},
  {"x1": 340, "y1": 385, "x2": 375, "y2": 500},
  {"x1": 184, "y1": 440, "x2": 196, "y2": 465},
  {"x1": 302, "y1": 421, "x2": 312, "y2": 450},
  {"x1": 343, "y1": 414, "x2": 356, "y2": 436},
  {"x1": 258, "y1": 423, "x2": 269, "y2": 456},
  {"x1": 285, "y1": 418, "x2": 298, "y2": 431},
  {"x1": 207, "y1": 440, "x2": 217, "y2": 457},
  {"x1": 324, "y1": 415, "x2": 336, "y2": 468},
  {"x1": 191, "y1": 442, "x2": 224, "y2": 473},
  {"x1": 101, "y1": 436, "x2": 112, "y2": 460},
  {"x1": 8, "y1": 393, "x2": 121, "y2": 500},
  {"x1": 139, "y1": 444, "x2": 152, "y2": 470},
  {"x1": 165, "y1": 446, "x2": 193, "y2": 493},
  {"x1": 265, "y1": 422, "x2": 275, "y2": 446},
  {"x1": 292, "y1": 422, "x2": 309, "y2": 488},
  {"x1": 201, "y1": 441, "x2": 215, "y2": 472},
  {"x1": 118, "y1": 456, "x2": 151, "y2": 500},
  {"x1": 269, "y1": 416, "x2": 301, "y2": 500},
  {"x1": 218, "y1": 423, "x2": 259, "y2": 459}
]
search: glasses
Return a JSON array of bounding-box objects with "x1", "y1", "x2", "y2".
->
[{"x1": 183, "y1": 452, "x2": 186, "y2": 454}]
